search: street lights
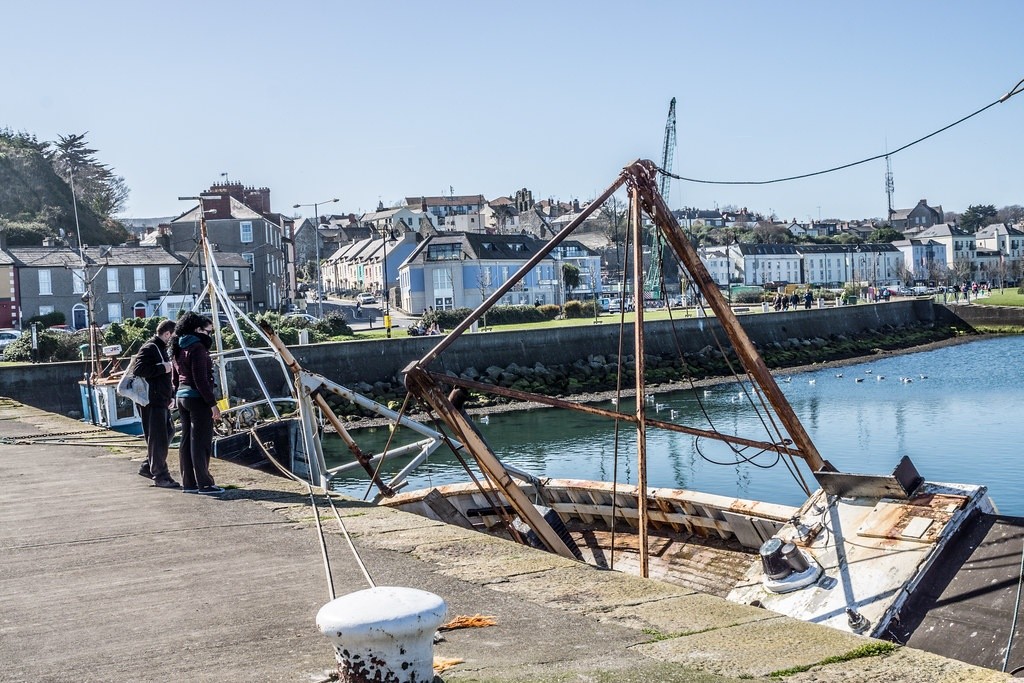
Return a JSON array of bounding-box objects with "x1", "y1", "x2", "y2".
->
[
  {"x1": 292, "y1": 198, "x2": 339, "y2": 320},
  {"x1": 382, "y1": 222, "x2": 398, "y2": 315}
]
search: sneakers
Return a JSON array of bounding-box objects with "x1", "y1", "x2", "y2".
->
[
  {"x1": 155, "y1": 476, "x2": 180, "y2": 488},
  {"x1": 182, "y1": 485, "x2": 199, "y2": 493},
  {"x1": 137, "y1": 467, "x2": 155, "y2": 480},
  {"x1": 198, "y1": 485, "x2": 225, "y2": 494}
]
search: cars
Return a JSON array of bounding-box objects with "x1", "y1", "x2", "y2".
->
[
  {"x1": 315, "y1": 291, "x2": 328, "y2": 301},
  {"x1": 595, "y1": 298, "x2": 616, "y2": 311},
  {"x1": 357, "y1": 293, "x2": 375, "y2": 305},
  {"x1": 51, "y1": 325, "x2": 77, "y2": 333},
  {"x1": 297, "y1": 283, "x2": 308, "y2": 291},
  {"x1": 676, "y1": 295, "x2": 689, "y2": 306},
  {"x1": 289, "y1": 314, "x2": 320, "y2": 329},
  {"x1": 0, "y1": 329, "x2": 25, "y2": 351},
  {"x1": 609, "y1": 298, "x2": 628, "y2": 314},
  {"x1": 890, "y1": 286, "x2": 955, "y2": 294}
]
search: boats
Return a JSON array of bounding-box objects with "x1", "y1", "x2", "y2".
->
[
  {"x1": 77, "y1": 196, "x2": 320, "y2": 488},
  {"x1": 257, "y1": 157, "x2": 1024, "y2": 680}
]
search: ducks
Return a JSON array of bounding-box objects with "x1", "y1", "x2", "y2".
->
[
  {"x1": 784, "y1": 369, "x2": 928, "y2": 386},
  {"x1": 481, "y1": 415, "x2": 489, "y2": 420},
  {"x1": 645, "y1": 388, "x2": 755, "y2": 419},
  {"x1": 611, "y1": 397, "x2": 617, "y2": 404}
]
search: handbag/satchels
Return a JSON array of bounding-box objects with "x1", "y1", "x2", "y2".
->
[{"x1": 117, "y1": 343, "x2": 165, "y2": 407}]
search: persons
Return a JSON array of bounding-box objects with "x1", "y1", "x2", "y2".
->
[
  {"x1": 172, "y1": 311, "x2": 225, "y2": 494},
  {"x1": 868, "y1": 285, "x2": 891, "y2": 303},
  {"x1": 772, "y1": 287, "x2": 814, "y2": 311},
  {"x1": 448, "y1": 384, "x2": 488, "y2": 446},
  {"x1": 357, "y1": 301, "x2": 363, "y2": 318},
  {"x1": 954, "y1": 280, "x2": 991, "y2": 301},
  {"x1": 626, "y1": 295, "x2": 636, "y2": 312},
  {"x1": 410, "y1": 306, "x2": 442, "y2": 335},
  {"x1": 133, "y1": 320, "x2": 180, "y2": 488},
  {"x1": 841, "y1": 290, "x2": 850, "y2": 305}
]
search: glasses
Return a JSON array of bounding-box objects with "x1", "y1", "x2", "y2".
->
[{"x1": 203, "y1": 329, "x2": 211, "y2": 336}]
